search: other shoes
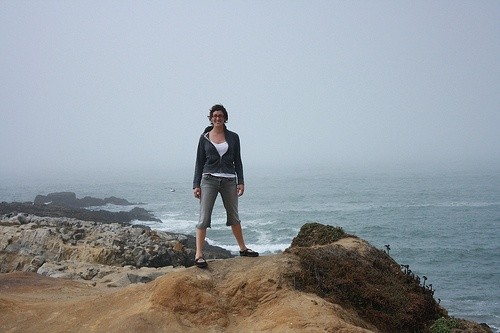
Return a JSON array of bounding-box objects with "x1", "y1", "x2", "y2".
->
[
  {"x1": 194, "y1": 257, "x2": 207, "y2": 268},
  {"x1": 240, "y1": 249, "x2": 259, "y2": 257}
]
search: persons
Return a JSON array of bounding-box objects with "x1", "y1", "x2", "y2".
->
[{"x1": 191, "y1": 104, "x2": 259, "y2": 268}]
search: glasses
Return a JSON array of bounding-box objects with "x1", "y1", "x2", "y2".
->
[{"x1": 212, "y1": 114, "x2": 224, "y2": 118}]
